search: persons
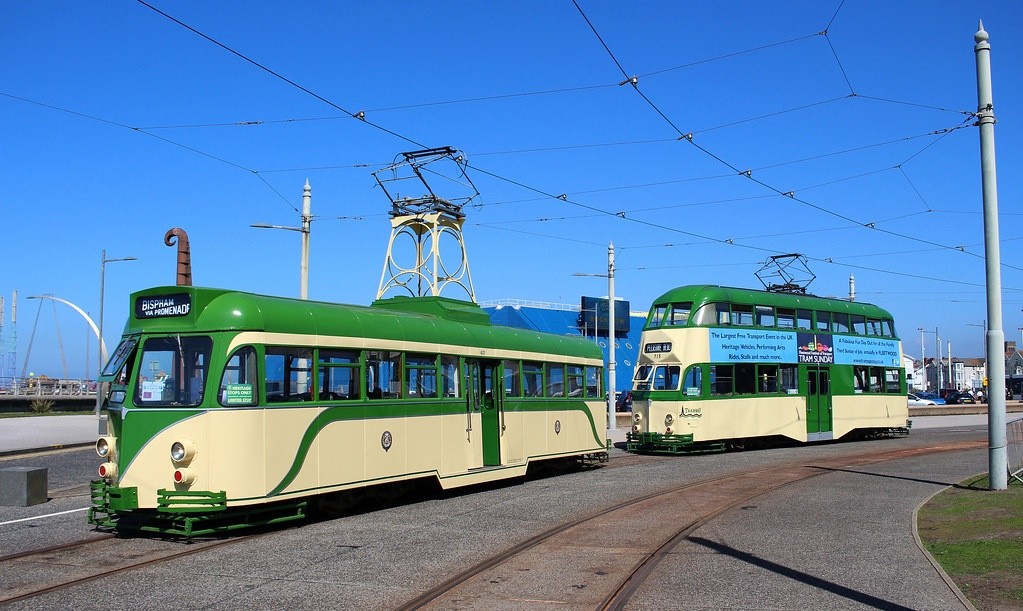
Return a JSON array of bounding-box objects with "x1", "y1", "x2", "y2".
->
[
  {"x1": 1010, "y1": 389, "x2": 1013, "y2": 400},
  {"x1": 968, "y1": 389, "x2": 973, "y2": 396},
  {"x1": 977, "y1": 389, "x2": 983, "y2": 400},
  {"x1": 1006, "y1": 387, "x2": 1008, "y2": 399}
]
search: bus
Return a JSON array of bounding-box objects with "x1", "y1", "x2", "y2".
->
[
  {"x1": 623, "y1": 252, "x2": 912, "y2": 457},
  {"x1": 89, "y1": 147, "x2": 612, "y2": 544}
]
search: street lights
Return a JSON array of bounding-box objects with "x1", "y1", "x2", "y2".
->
[
  {"x1": 572, "y1": 246, "x2": 618, "y2": 449},
  {"x1": 966, "y1": 319, "x2": 987, "y2": 385},
  {"x1": 919, "y1": 327, "x2": 941, "y2": 398},
  {"x1": 248, "y1": 173, "x2": 312, "y2": 302},
  {"x1": 95, "y1": 249, "x2": 136, "y2": 420}
]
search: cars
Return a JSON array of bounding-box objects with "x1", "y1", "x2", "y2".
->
[
  {"x1": 483, "y1": 387, "x2": 634, "y2": 412},
  {"x1": 907, "y1": 388, "x2": 1014, "y2": 405}
]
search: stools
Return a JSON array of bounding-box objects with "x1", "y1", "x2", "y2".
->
[{"x1": 0, "y1": 466, "x2": 49, "y2": 507}]
